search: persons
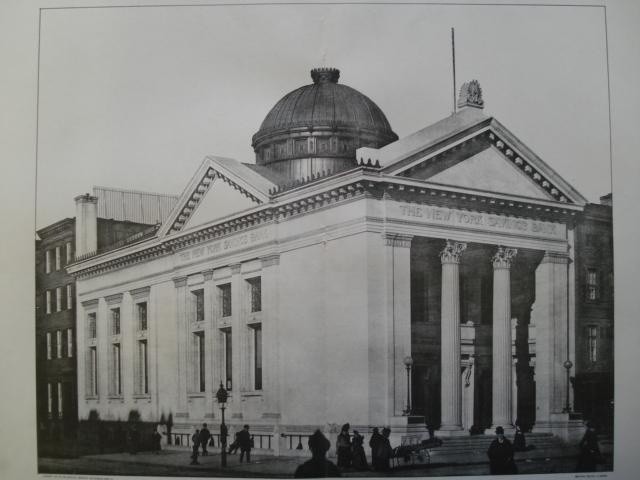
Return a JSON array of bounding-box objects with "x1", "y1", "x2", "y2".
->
[
  {"x1": 295, "y1": 430, "x2": 342, "y2": 478},
  {"x1": 239, "y1": 424, "x2": 251, "y2": 464},
  {"x1": 192, "y1": 423, "x2": 210, "y2": 456},
  {"x1": 575, "y1": 423, "x2": 600, "y2": 472},
  {"x1": 335, "y1": 423, "x2": 394, "y2": 472},
  {"x1": 487, "y1": 426, "x2": 518, "y2": 474}
]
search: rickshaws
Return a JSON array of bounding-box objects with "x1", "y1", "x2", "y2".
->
[{"x1": 391, "y1": 435, "x2": 443, "y2": 466}]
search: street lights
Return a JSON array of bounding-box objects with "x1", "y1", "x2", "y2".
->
[{"x1": 216, "y1": 379, "x2": 228, "y2": 467}]
